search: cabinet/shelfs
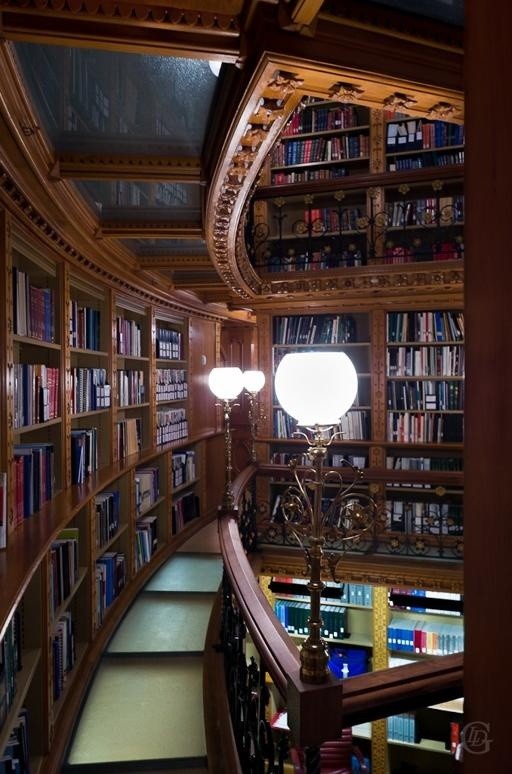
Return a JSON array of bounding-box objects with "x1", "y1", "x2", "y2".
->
[
  {"x1": 253, "y1": 100, "x2": 464, "y2": 272},
  {"x1": 275, "y1": 596, "x2": 464, "y2": 756},
  {"x1": 0, "y1": 209, "x2": 206, "y2": 774},
  {"x1": 257, "y1": 293, "x2": 464, "y2": 548}
]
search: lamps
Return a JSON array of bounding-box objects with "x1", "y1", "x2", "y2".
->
[
  {"x1": 272, "y1": 352, "x2": 358, "y2": 685},
  {"x1": 244, "y1": 371, "x2": 266, "y2": 465},
  {"x1": 209, "y1": 367, "x2": 245, "y2": 516}
]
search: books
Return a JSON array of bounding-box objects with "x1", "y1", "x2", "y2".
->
[{"x1": 0, "y1": 44, "x2": 466, "y2": 772}]
